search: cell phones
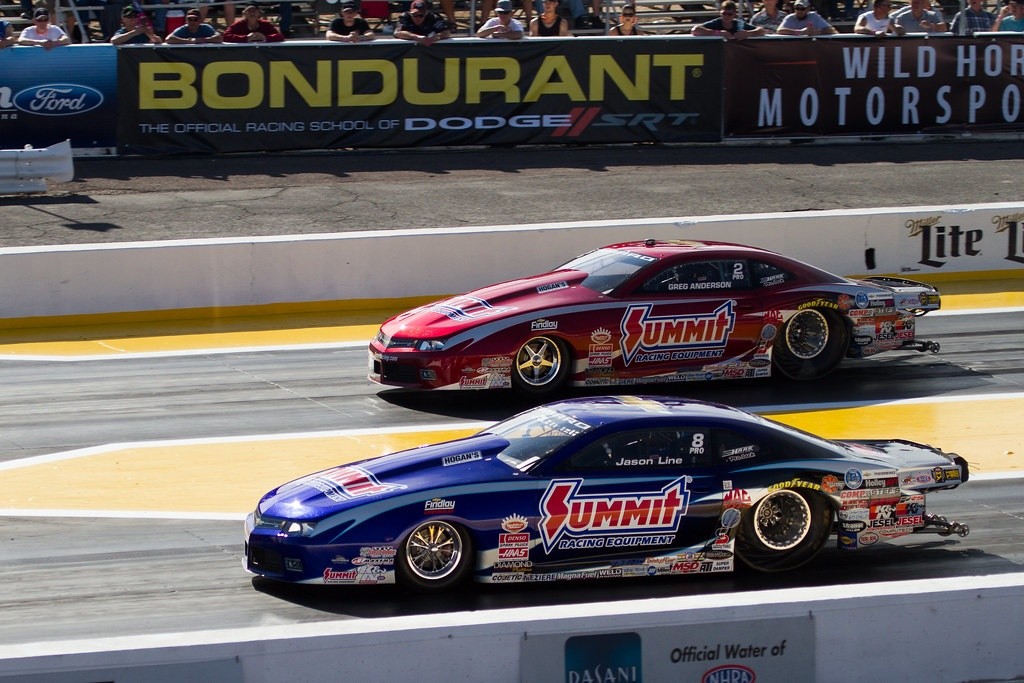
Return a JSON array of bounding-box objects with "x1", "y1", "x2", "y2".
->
[{"x1": 140, "y1": 17, "x2": 147, "y2": 28}]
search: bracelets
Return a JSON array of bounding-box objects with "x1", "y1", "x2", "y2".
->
[
  {"x1": 795, "y1": 29, "x2": 801, "y2": 35},
  {"x1": 873, "y1": 30, "x2": 876, "y2": 35},
  {"x1": 930, "y1": 23, "x2": 934, "y2": 30},
  {"x1": 999, "y1": 15, "x2": 1004, "y2": 19},
  {"x1": 715, "y1": 30, "x2": 719, "y2": 35}
]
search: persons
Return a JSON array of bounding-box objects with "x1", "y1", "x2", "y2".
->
[
  {"x1": 477, "y1": 0, "x2": 523, "y2": 40},
  {"x1": 165, "y1": 5, "x2": 284, "y2": 43},
  {"x1": 529, "y1": 0, "x2": 574, "y2": 37},
  {"x1": 609, "y1": 4, "x2": 644, "y2": 36},
  {"x1": 441, "y1": 0, "x2": 493, "y2": 33},
  {"x1": 0, "y1": 0, "x2": 91, "y2": 49},
  {"x1": 777, "y1": 0, "x2": 839, "y2": 35},
  {"x1": 749, "y1": 0, "x2": 788, "y2": 34},
  {"x1": 110, "y1": 6, "x2": 163, "y2": 45},
  {"x1": 854, "y1": 0, "x2": 1024, "y2": 37},
  {"x1": 691, "y1": 0, "x2": 765, "y2": 40},
  {"x1": 812, "y1": 0, "x2": 854, "y2": 21},
  {"x1": 195, "y1": 0, "x2": 234, "y2": 26},
  {"x1": 326, "y1": 0, "x2": 374, "y2": 41},
  {"x1": 525, "y1": 0, "x2": 606, "y2": 30},
  {"x1": 280, "y1": 1, "x2": 294, "y2": 37},
  {"x1": 394, "y1": 0, "x2": 451, "y2": 46}
]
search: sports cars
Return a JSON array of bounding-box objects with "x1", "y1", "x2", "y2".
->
[
  {"x1": 367, "y1": 237, "x2": 942, "y2": 403},
  {"x1": 241, "y1": 394, "x2": 971, "y2": 607}
]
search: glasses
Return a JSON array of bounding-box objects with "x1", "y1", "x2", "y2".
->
[
  {"x1": 623, "y1": 13, "x2": 634, "y2": 17},
  {"x1": 722, "y1": 12, "x2": 735, "y2": 16},
  {"x1": 35, "y1": 18, "x2": 49, "y2": 23},
  {"x1": 188, "y1": 17, "x2": 198, "y2": 21},
  {"x1": 795, "y1": 7, "x2": 807, "y2": 11},
  {"x1": 498, "y1": 11, "x2": 511, "y2": 15},
  {"x1": 412, "y1": 13, "x2": 426, "y2": 18},
  {"x1": 880, "y1": 4, "x2": 891, "y2": 8}
]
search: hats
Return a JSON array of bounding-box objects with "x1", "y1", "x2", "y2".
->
[
  {"x1": 494, "y1": 0, "x2": 512, "y2": 11},
  {"x1": 409, "y1": 0, "x2": 428, "y2": 13},
  {"x1": 342, "y1": 1, "x2": 357, "y2": 11},
  {"x1": 187, "y1": 9, "x2": 201, "y2": 19},
  {"x1": 123, "y1": 6, "x2": 141, "y2": 16},
  {"x1": 242, "y1": 6, "x2": 259, "y2": 15},
  {"x1": 33, "y1": 8, "x2": 49, "y2": 19},
  {"x1": 794, "y1": 0, "x2": 810, "y2": 7}
]
